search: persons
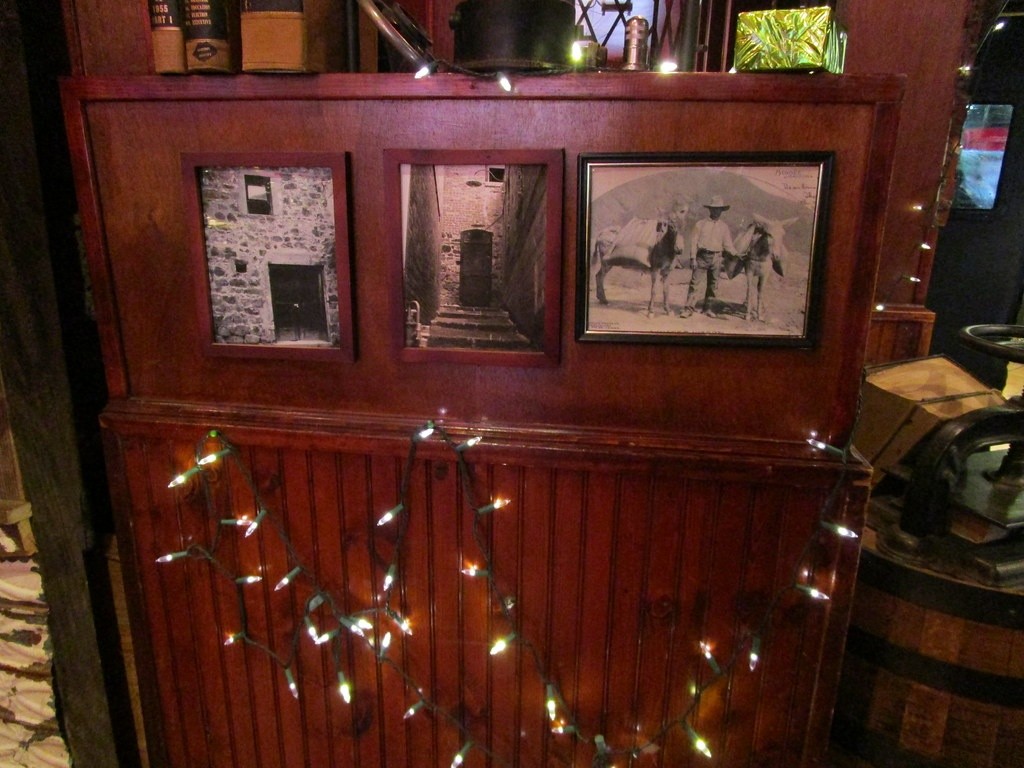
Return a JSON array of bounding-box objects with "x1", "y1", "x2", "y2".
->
[{"x1": 678, "y1": 195, "x2": 747, "y2": 318}]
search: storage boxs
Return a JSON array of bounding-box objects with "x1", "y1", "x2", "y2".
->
[{"x1": 735, "y1": 6, "x2": 848, "y2": 74}]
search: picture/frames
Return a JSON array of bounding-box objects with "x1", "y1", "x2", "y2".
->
[
  {"x1": 575, "y1": 149, "x2": 834, "y2": 352},
  {"x1": 181, "y1": 150, "x2": 355, "y2": 365},
  {"x1": 383, "y1": 145, "x2": 565, "y2": 366}
]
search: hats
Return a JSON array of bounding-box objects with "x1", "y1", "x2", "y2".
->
[{"x1": 702, "y1": 194, "x2": 730, "y2": 211}]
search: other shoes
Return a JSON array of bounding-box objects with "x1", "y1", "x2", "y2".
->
[
  {"x1": 705, "y1": 310, "x2": 716, "y2": 317},
  {"x1": 680, "y1": 308, "x2": 692, "y2": 318}
]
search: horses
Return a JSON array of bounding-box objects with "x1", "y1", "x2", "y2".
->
[
  {"x1": 690, "y1": 213, "x2": 800, "y2": 322},
  {"x1": 592, "y1": 204, "x2": 688, "y2": 318}
]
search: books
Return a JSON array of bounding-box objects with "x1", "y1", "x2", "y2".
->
[{"x1": 149, "y1": 0, "x2": 346, "y2": 75}]
